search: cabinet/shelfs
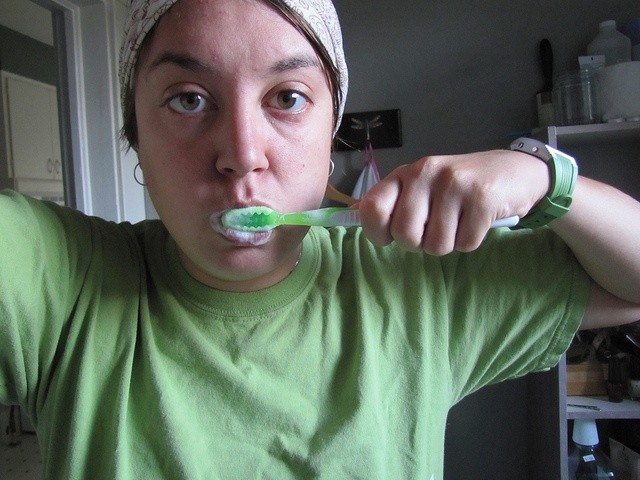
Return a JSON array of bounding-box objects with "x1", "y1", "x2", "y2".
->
[
  {"x1": 0, "y1": 69, "x2": 65, "y2": 199},
  {"x1": 529, "y1": 121, "x2": 640, "y2": 480}
]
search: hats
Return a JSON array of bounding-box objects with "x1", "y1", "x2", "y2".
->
[{"x1": 118, "y1": 0, "x2": 349, "y2": 153}]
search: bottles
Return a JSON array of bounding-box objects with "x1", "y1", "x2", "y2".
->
[
  {"x1": 552, "y1": 71, "x2": 601, "y2": 127},
  {"x1": 568, "y1": 419, "x2": 623, "y2": 480},
  {"x1": 586, "y1": 18, "x2": 632, "y2": 66}
]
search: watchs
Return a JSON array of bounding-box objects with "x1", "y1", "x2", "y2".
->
[{"x1": 505, "y1": 135, "x2": 577, "y2": 231}]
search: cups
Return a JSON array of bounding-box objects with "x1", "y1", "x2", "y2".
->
[{"x1": 578, "y1": 55, "x2": 607, "y2": 123}]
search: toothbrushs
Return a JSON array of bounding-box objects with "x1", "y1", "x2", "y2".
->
[{"x1": 219, "y1": 204, "x2": 521, "y2": 233}]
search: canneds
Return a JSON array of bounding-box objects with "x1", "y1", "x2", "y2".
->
[{"x1": 556, "y1": 72, "x2": 599, "y2": 125}]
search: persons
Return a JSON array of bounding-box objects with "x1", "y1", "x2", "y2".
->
[{"x1": 3, "y1": 2, "x2": 640, "y2": 479}]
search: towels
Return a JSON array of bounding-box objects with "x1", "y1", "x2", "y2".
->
[{"x1": 350, "y1": 143, "x2": 381, "y2": 202}]
